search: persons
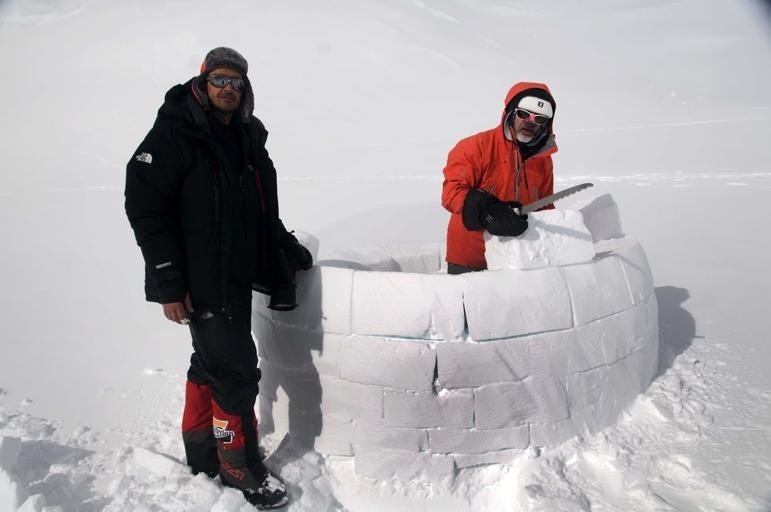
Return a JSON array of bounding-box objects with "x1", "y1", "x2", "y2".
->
[
  {"x1": 124, "y1": 46, "x2": 312, "y2": 509},
  {"x1": 442, "y1": 83, "x2": 558, "y2": 274}
]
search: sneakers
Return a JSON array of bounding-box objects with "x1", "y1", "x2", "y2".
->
[{"x1": 221, "y1": 465, "x2": 288, "y2": 508}]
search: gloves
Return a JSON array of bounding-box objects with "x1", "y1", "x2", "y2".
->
[
  {"x1": 253, "y1": 229, "x2": 312, "y2": 309},
  {"x1": 461, "y1": 187, "x2": 527, "y2": 236}
]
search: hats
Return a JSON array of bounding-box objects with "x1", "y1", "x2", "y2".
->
[{"x1": 192, "y1": 47, "x2": 254, "y2": 123}]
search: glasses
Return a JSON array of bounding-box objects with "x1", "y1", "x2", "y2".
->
[
  {"x1": 514, "y1": 107, "x2": 548, "y2": 125},
  {"x1": 205, "y1": 74, "x2": 244, "y2": 91}
]
